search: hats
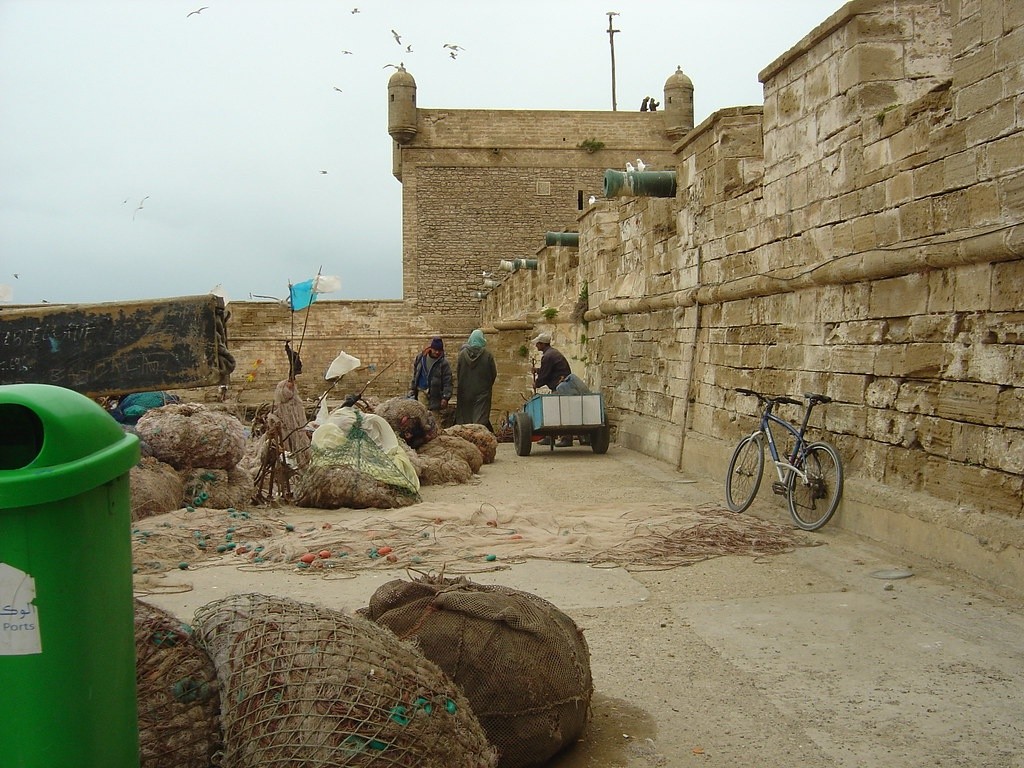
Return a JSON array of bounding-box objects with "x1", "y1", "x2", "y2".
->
[
  {"x1": 531, "y1": 333, "x2": 551, "y2": 344},
  {"x1": 431, "y1": 338, "x2": 444, "y2": 350}
]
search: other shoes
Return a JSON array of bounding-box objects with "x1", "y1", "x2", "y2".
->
[
  {"x1": 537, "y1": 437, "x2": 555, "y2": 445},
  {"x1": 555, "y1": 439, "x2": 573, "y2": 446}
]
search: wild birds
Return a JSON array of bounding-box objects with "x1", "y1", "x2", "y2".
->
[
  {"x1": 333, "y1": 86, "x2": 342, "y2": 92},
  {"x1": 443, "y1": 43, "x2": 466, "y2": 59},
  {"x1": 186, "y1": 6, "x2": 209, "y2": 17},
  {"x1": 319, "y1": 170, "x2": 328, "y2": 174},
  {"x1": 13, "y1": 273, "x2": 19, "y2": 279},
  {"x1": 341, "y1": 50, "x2": 352, "y2": 54},
  {"x1": 351, "y1": 8, "x2": 360, "y2": 14},
  {"x1": 392, "y1": 29, "x2": 401, "y2": 45},
  {"x1": 121, "y1": 195, "x2": 150, "y2": 221},
  {"x1": 405, "y1": 44, "x2": 414, "y2": 53},
  {"x1": 382, "y1": 63, "x2": 399, "y2": 69}
]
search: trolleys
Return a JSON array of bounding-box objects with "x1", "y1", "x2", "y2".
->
[{"x1": 513, "y1": 394, "x2": 610, "y2": 456}]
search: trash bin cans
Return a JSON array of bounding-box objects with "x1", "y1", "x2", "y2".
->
[{"x1": 0, "y1": 383, "x2": 143, "y2": 768}]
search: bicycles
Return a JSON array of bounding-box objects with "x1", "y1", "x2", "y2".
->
[{"x1": 726, "y1": 388, "x2": 844, "y2": 531}]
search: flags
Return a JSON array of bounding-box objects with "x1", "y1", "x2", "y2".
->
[
  {"x1": 312, "y1": 275, "x2": 340, "y2": 293},
  {"x1": 289, "y1": 279, "x2": 318, "y2": 311},
  {"x1": 326, "y1": 351, "x2": 360, "y2": 380}
]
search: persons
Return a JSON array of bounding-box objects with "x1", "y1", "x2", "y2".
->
[
  {"x1": 649, "y1": 98, "x2": 659, "y2": 111},
  {"x1": 456, "y1": 329, "x2": 497, "y2": 433},
  {"x1": 409, "y1": 337, "x2": 453, "y2": 428},
  {"x1": 640, "y1": 97, "x2": 650, "y2": 112},
  {"x1": 531, "y1": 334, "x2": 573, "y2": 448}
]
